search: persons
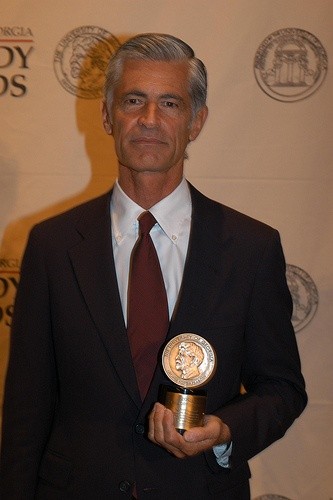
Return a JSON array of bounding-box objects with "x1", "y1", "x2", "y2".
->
[{"x1": 0, "y1": 32, "x2": 308, "y2": 500}]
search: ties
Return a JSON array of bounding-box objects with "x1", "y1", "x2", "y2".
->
[{"x1": 122, "y1": 208, "x2": 171, "y2": 400}]
